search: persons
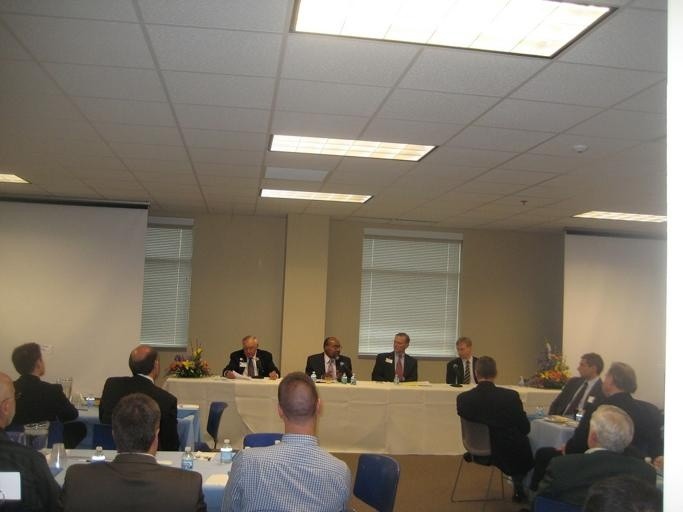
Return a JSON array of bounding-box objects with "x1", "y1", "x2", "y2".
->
[
  {"x1": 0, "y1": 372, "x2": 63, "y2": 512},
  {"x1": 63, "y1": 393, "x2": 208, "y2": 511},
  {"x1": 583, "y1": 475, "x2": 663, "y2": 512},
  {"x1": 222, "y1": 335, "x2": 280, "y2": 380},
  {"x1": 7, "y1": 342, "x2": 87, "y2": 447},
  {"x1": 221, "y1": 371, "x2": 352, "y2": 512},
  {"x1": 100, "y1": 344, "x2": 181, "y2": 451},
  {"x1": 519, "y1": 404, "x2": 657, "y2": 511},
  {"x1": 372, "y1": 332, "x2": 417, "y2": 382},
  {"x1": 446, "y1": 336, "x2": 478, "y2": 384},
  {"x1": 529, "y1": 361, "x2": 662, "y2": 491},
  {"x1": 548, "y1": 353, "x2": 603, "y2": 421},
  {"x1": 457, "y1": 356, "x2": 534, "y2": 501},
  {"x1": 306, "y1": 336, "x2": 352, "y2": 380}
]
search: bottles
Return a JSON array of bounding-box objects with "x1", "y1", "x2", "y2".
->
[
  {"x1": 341, "y1": 372, "x2": 347, "y2": 384},
  {"x1": 219, "y1": 438, "x2": 232, "y2": 463},
  {"x1": 393, "y1": 374, "x2": 399, "y2": 384},
  {"x1": 49, "y1": 442, "x2": 68, "y2": 485},
  {"x1": 86, "y1": 394, "x2": 96, "y2": 416},
  {"x1": 310, "y1": 371, "x2": 317, "y2": 383},
  {"x1": 90, "y1": 446, "x2": 107, "y2": 465},
  {"x1": 349, "y1": 374, "x2": 357, "y2": 385},
  {"x1": 180, "y1": 447, "x2": 194, "y2": 472}
]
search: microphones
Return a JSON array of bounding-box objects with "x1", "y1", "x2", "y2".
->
[
  {"x1": 453, "y1": 363, "x2": 462, "y2": 377},
  {"x1": 340, "y1": 362, "x2": 351, "y2": 375}
]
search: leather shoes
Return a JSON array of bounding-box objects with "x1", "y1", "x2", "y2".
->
[{"x1": 513, "y1": 491, "x2": 530, "y2": 504}]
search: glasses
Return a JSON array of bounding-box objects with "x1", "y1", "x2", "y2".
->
[
  {"x1": 333, "y1": 345, "x2": 343, "y2": 351},
  {"x1": 1, "y1": 391, "x2": 23, "y2": 405}
]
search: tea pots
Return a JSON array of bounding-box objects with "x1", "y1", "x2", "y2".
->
[
  {"x1": 55, "y1": 376, "x2": 74, "y2": 402},
  {"x1": 23, "y1": 420, "x2": 49, "y2": 459}
]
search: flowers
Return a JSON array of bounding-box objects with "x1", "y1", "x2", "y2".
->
[
  {"x1": 523, "y1": 336, "x2": 572, "y2": 390},
  {"x1": 160, "y1": 336, "x2": 212, "y2": 378}
]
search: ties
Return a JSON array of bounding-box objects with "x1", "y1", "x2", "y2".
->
[
  {"x1": 396, "y1": 355, "x2": 402, "y2": 382},
  {"x1": 464, "y1": 361, "x2": 470, "y2": 384},
  {"x1": 564, "y1": 382, "x2": 588, "y2": 416},
  {"x1": 248, "y1": 358, "x2": 256, "y2": 378},
  {"x1": 328, "y1": 359, "x2": 334, "y2": 380}
]
